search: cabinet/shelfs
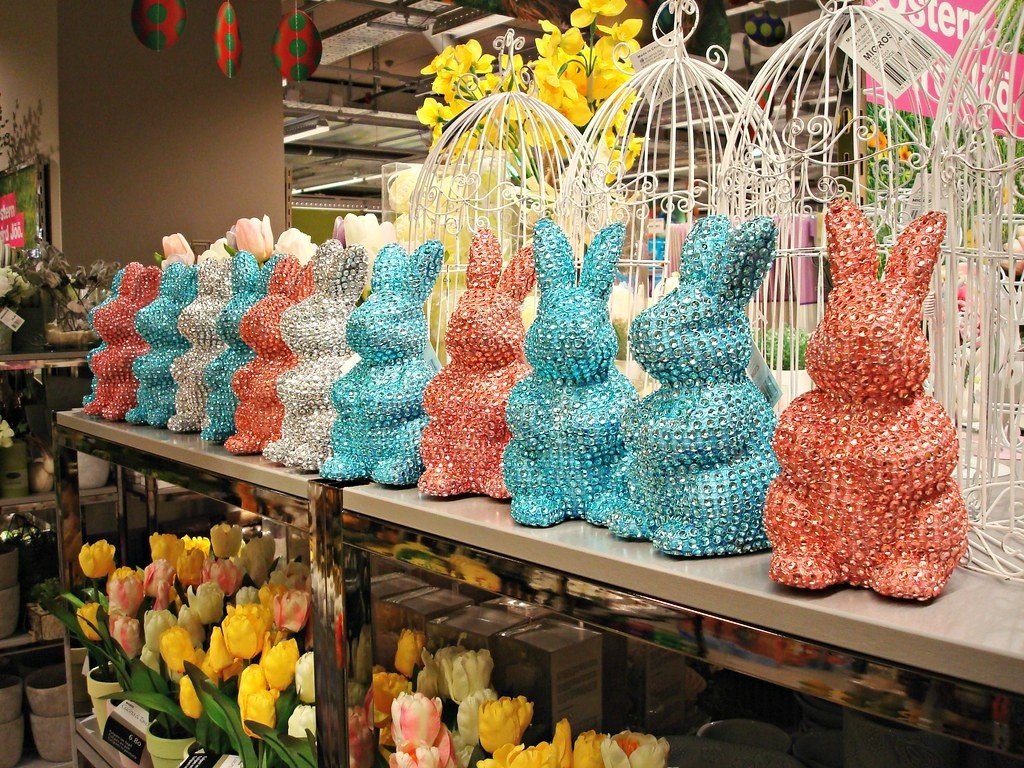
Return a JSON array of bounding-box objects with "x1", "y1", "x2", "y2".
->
[
  {"x1": 50, "y1": 407, "x2": 374, "y2": 768},
  {"x1": 1, "y1": 351, "x2": 127, "y2": 768},
  {"x1": 123, "y1": 464, "x2": 262, "y2": 570},
  {"x1": 327, "y1": 476, "x2": 1024, "y2": 767}
]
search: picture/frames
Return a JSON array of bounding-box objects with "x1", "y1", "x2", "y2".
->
[{"x1": 0, "y1": 153, "x2": 51, "y2": 261}]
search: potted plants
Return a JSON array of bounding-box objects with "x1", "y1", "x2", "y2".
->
[
  {"x1": 0, "y1": 266, "x2": 46, "y2": 352},
  {"x1": 0, "y1": 437, "x2": 30, "y2": 497},
  {"x1": 610, "y1": 318, "x2": 818, "y2": 415},
  {"x1": 25, "y1": 575, "x2": 66, "y2": 641}
]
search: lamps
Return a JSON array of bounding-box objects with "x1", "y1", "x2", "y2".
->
[
  {"x1": 284, "y1": 114, "x2": 330, "y2": 143},
  {"x1": 725, "y1": 0, "x2": 788, "y2": 16},
  {"x1": 431, "y1": 7, "x2": 516, "y2": 39},
  {"x1": 292, "y1": 78, "x2": 839, "y2": 197}
]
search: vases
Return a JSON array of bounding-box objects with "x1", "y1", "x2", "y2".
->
[
  {"x1": 0, "y1": 306, "x2": 19, "y2": 354},
  {"x1": 0, "y1": 581, "x2": 21, "y2": 640},
  {"x1": 183, "y1": 741, "x2": 240, "y2": 768},
  {"x1": 18, "y1": 655, "x2": 47, "y2": 679},
  {"x1": 78, "y1": 450, "x2": 110, "y2": 489},
  {"x1": 0, "y1": 542, "x2": 18, "y2": 591},
  {"x1": 118, "y1": 723, "x2": 154, "y2": 768},
  {"x1": 685, "y1": 656, "x2": 1024, "y2": 768},
  {"x1": 86, "y1": 661, "x2": 125, "y2": 736},
  {"x1": 27, "y1": 706, "x2": 74, "y2": 764},
  {"x1": 0, "y1": 673, "x2": 22, "y2": 723},
  {"x1": 146, "y1": 719, "x2": 197, "y2": 768},
  {"x1": 46, "y1": 322, "x2": 99, "y2": 352},
  {"x1": 25, "y1": 662, "x2": 69, "y2": 717},
  {"x1": 1, "y1": 714, "x2": 25, "y2": 768},
  {"x1": 27, "y1": 454, "x2": 56, "y2": 493}
]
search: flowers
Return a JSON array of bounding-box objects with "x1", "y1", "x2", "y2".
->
[
  {"x1": 932, "y1": 225, "x2": 1024, "y2": 430},
  {"x1": 154, "y1": 0, "x2": 681, "y2": 365},
  {"x1": 0, "y1": 420, "x2": 29, "y2": 449},
  {"x1": 30, "y1": 523, "x2": 672, "y2": 768},
  {"x1": 33, "y1": 235, "x2": 122, "y2": 331},
  {"x1": 0, "y1": 274, "x2": 12, "y2": 309}
]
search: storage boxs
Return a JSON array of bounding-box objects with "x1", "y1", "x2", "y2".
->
[
  {"x1": 426, "y1": 605, "x2": 531, "y2": 660},
  {"x1": 375, "y1": 585, "x2": 475, "y2": 672},
  {"x1": 370, "y1": 568, "x2": 432, "y2": 632},
  {"x1": 582, "y1": 621, "x2": 684, "y2": 736},
  {"x1": 481, "y1": 595, "x2": 554, "y2": 622},
  {"x1": 493, "y1": 617, "x2": 603, "y2": 750}
]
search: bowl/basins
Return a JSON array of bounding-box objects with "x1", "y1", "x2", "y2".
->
[
  {"x1": 0, "y1": 547, "x2": 72, "y2": 768},
  {"x1": 697, "y1": 685, "x2": 843, "y2": 768}
]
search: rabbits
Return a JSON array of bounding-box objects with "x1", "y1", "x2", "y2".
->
[
  {"x1": 225, "y1": 254, "x2": 315, "y2": 454},
  {"x1": 611, "y1": 214, "x2": 783, "y2": 558},
  {"x1": 83, "y1": 263, "x2": 162, "y2": 420},
  {"x1": 82, "y1": 269, "x2": 122, "y2": 408},
  {"x1": 167, "y1": 260, "x2": 233, "y2": 432},
  {"x1": 124, "y1": 262, "x2": 198, "y2": 427},
  {"x1": 200, "y1": 251, "x2": 286, "y2": 441},
  {"x1": 503, "y1": 217, "x2": 640, "y2": 527},
  {"x1": 321, "y1": 240, "x2": 436, "y2": 485},
  {"x1": 419, "y1": 228, "x2": 536, "y2": 499},
  {"x1": 262, "y1": 239, "x2": 367, "y2": 469},
  {"x1": 763, "y1": 197, "x2": 968, "y2": 603}
]
